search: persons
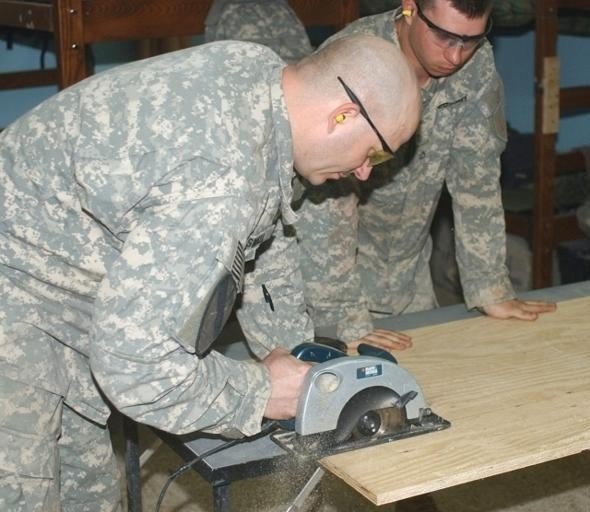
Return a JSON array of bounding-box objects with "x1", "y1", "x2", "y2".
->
[
  {"x1": 0, "y1": 33, "x2": 423, "y2": 512},
  {"x1": 205, "y1": 2, "x2": 558, "y2": 351}
]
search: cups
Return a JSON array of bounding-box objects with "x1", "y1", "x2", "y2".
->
[
  {"x1": 334, "y1": 75, "x2": 395, "y2": 168},
  {"x1": 415, "y1": 1, "x2": 493, "y2": 49}
]
